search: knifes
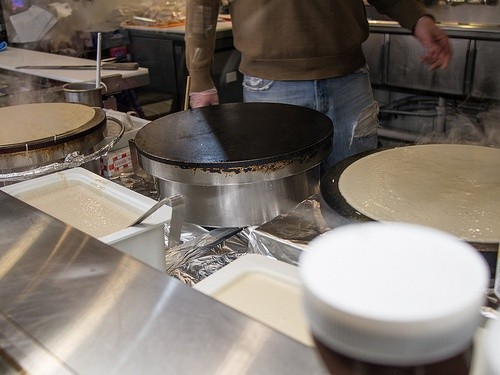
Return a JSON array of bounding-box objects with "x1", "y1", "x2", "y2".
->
[{"x1": 16, "y1": 63, "x2": 138, "y2": 70}]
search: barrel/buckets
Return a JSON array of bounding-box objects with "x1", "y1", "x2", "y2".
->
[{"x1": 62, "y1": 81, "x2": 104, "y2": 108}]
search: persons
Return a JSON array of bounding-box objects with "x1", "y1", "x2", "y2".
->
[{"x1": 182, "y1": 0, "x2": 453, "y2": 179}]
